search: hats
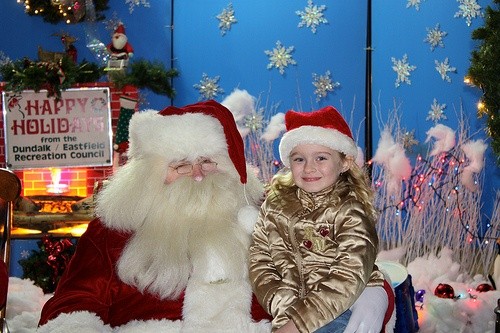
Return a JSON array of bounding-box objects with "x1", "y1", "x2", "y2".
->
[
  {"x1": 278, "y1": 105, "x2": 357, "y2": 168},
  {"x1": 127, "y1": 99, "x2": 259, "y2": 235}
]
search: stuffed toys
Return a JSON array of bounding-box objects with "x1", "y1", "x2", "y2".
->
[{"x1": 104, "y1": 24, "x2": 134, "y2": 60}]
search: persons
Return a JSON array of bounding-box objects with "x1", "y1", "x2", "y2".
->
[
  {"x1": 250, "y1": 106, "x2": 384, "y2": 333},
  {"x1": 36, "y1": 99, "x2": 395, "y2": 333}
]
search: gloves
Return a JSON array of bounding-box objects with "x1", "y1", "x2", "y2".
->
[{"x1": 343, "y1": 286, "x2": 389, "y2": 333}]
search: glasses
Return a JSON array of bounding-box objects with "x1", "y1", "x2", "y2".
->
[{"x1": 168, "y1": 161, "x2": 218, "y2": 175}]
